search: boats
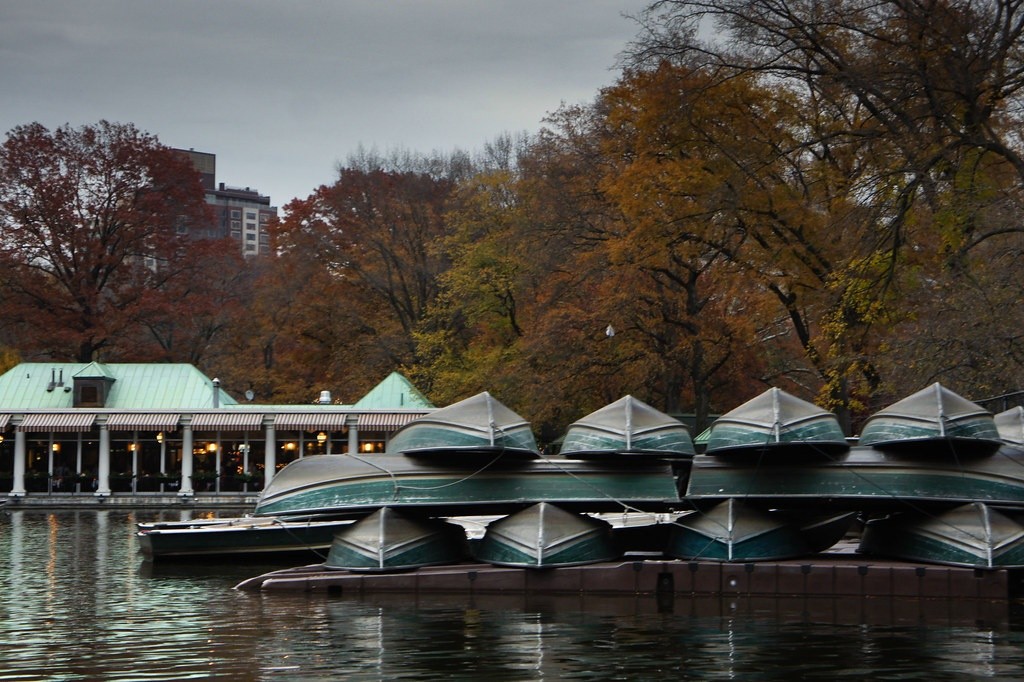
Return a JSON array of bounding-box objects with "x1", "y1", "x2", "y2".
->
[
  {"x1": 322, "y1": 505, "x2": 468, "y2": 573},
  {"x1": 472, "y1": 502, "x2": 625, "y2": 568},
  {"x1": 855, "y1": 382, "x2": 1004, "y2": 450},
  {"x1": 556, "y1": 398, "x2": 696, "y2": 460},
  {"x1": 853, "y1": 502, "x2": 1024, "y2": 570},
  {"x1": 664, "y1": 494, "x2": 817, "y2": 562},
  {"x1": 251, "y1": 443, "x2": 1024, "y2": 519},
  {"x1": 703, "y1": 385, "x2": 852, "y2": 456},
  {"x1": 387, "y1": 391, "x2": 542, "y2": 458}
]
